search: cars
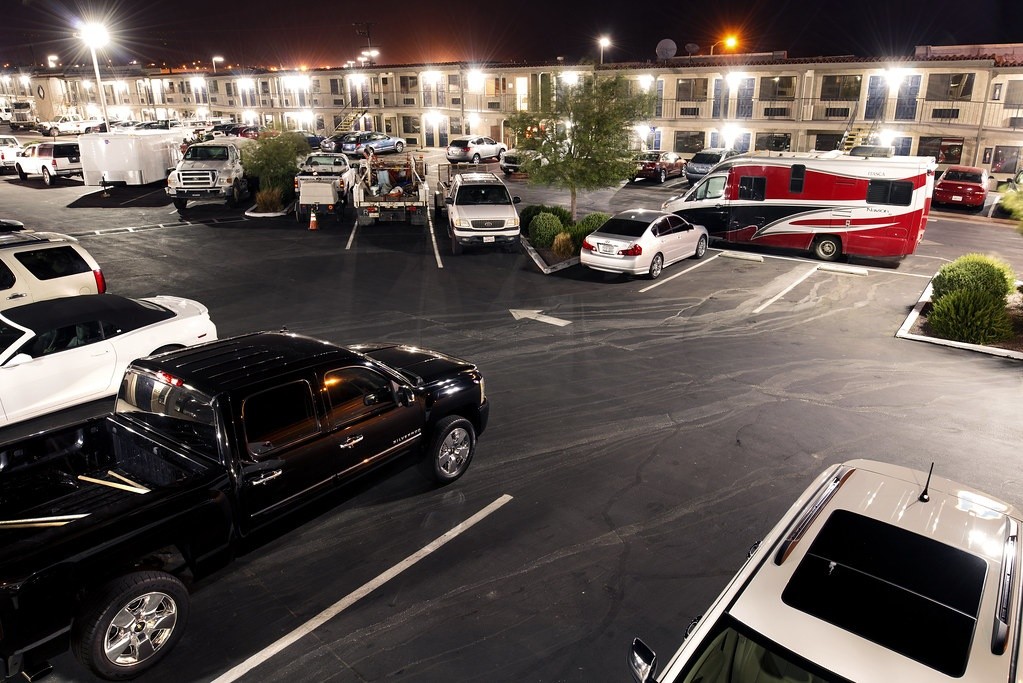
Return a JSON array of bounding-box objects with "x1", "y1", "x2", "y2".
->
[
  {"x1": 0, "y1": 107, "x2": 13, "y2": 125},
  {"x1": 0, "y1": 135, "x2": 23, "y2": 175},
  {"x1": 275, "y1": 130, "x2": 325, "y2": 149},
  {"x1": 319, "y1": 130, "x2": 360, "y2": 153},
  {"x1": 626, "y1": 150, "x2": 687, "y2": 184},
  {"x1": 627, "y1": 457, "x2": 1022, "y2": 683},
  {"x1": 89, "y1": 115, "x2": 179, "y2": 129},
  {"x1": 0, "y1": 219, "x2": 28, "y2": 235},
  {"x1": 15, "y1": 141, "x2": 85, "y2": 186},
  {"x1": 580, "y1": 208, "x2": 710, "y2": 280},
  {"x1": 934, "y1": 165, "x2": 995, "y2": 212},
  {"x1": 0, "y1": 292, "x2": 220, "y2": 427}
]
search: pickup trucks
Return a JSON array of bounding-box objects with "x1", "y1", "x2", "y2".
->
[
  {"x1": 165, "y1": 135, "x2": 262, "y2": 209},
  {"x1": 434, "y1": 171, "x2": 522, "y2": 256},
  {"x1": 0, "y1": 327, "x2": 490, "y2": 683},
  {"x1": 36, "y1": 113, "x2": 99, "y2": 137},
  {"x1": 294, "y1": 151, "x2": 358, "y2": 217}
]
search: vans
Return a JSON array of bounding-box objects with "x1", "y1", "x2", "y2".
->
[
  {"x1": 685, "y1": 148, "x2": 739, "y2": 183},
  {"x1": 848, "y1": 145, "x2": 897, "y2": 159},
  {"x1": 0, "y1": 230, "x2": 106, "y2": 312},
  {"x1": 660, "y1": 148, "x2": 936, "y2": 262}
]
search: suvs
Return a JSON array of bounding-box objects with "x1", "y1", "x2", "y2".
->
[
  {"x1": 499, "y1": 136, "x2": 569, "y2": 177},
  {"x1": 445, "y1": 135, "x2": 508, "y2": 166},
  {"x1": 341, "y1": 130, "x2": 406, "y2": 159}
]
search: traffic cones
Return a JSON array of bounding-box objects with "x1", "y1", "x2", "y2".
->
[{"x1": 307, "y1": 208, "x2": 321, "y2": 232}]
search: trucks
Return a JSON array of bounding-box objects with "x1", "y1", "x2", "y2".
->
[
  {"x1": 351, "y1": 151, "x2": 429, "y2": 226},
  {"x1": 9, "y1": 100, "x2": 36, "y2": 130}
]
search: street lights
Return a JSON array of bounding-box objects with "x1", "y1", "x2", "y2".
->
[
  {"x1": 83, "y1": 24, "x2": 111, "y2": 133},
  {"x1": 599, "y1": 35, "x2": 613, "y2": 64},
  {"x1": 710, "y1": 35, "x2": 737, "y2": 55},
  {"x1": 212, "y1": 56, "x2": 225, "y2": 73}
]
